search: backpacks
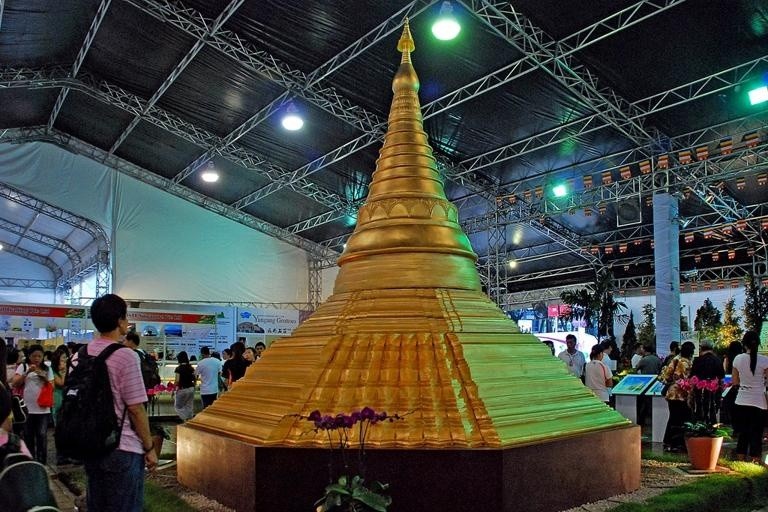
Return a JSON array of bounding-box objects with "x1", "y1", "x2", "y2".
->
[
  {"x1": 54, "y1": 342, "x2": 130, "y2": 466},
  {"x1": 0, "y1": 432, "x2": 61, "y2": 512},
  {"x1": 133, "y1": 347, "x2": 161, "y2": 389}
]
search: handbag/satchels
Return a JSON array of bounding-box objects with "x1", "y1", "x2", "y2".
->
[
  {"x1": 11, "y1": 394, "x2": 30, "y2": 425},
  {"x1": 37, "y1": 381, "x2": 55, "y2": 408},
  {"x1": 579, "y1": 374, "x2": 586, "y2": 386}
]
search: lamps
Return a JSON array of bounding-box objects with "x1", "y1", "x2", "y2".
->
[
  {"x1": 279, "y1": 99, "x2": 307, "y2": 133},
  {"x1": 429, "y1": 0, "x2": 462, "y2": 41},
  {"x1": 198, "y1": 158, "x2": 223, "y2": 185}
]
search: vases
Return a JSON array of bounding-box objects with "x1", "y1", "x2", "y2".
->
[
  {"x1": 679, "y1": 432, "x2": 735, "y2": 471},
  {"x1": 143, "y1": 431, "x2": 165, "y2": 459},
  {"x1": 315, "y1": 503, "x2": 330, "y2": 511}
]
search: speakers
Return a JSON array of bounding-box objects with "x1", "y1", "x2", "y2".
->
[{"x1": 614, "y1": 198, "x2": 640, "y2": 225}]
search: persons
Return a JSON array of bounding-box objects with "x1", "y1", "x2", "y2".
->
[{"x1": 542, "y1": 331, "x2": 768, "y2": 464}]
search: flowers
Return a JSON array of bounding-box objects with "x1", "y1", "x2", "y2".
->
[
  {"x1": 142, "y1": 381, "x2": 180, "y2": 441},
  {"x1": 673, "y1": 373, "x2": 736, "y2": 444},
  {"x1": 274, "y1": 402, "x2": 425, "y2": 512}
]
